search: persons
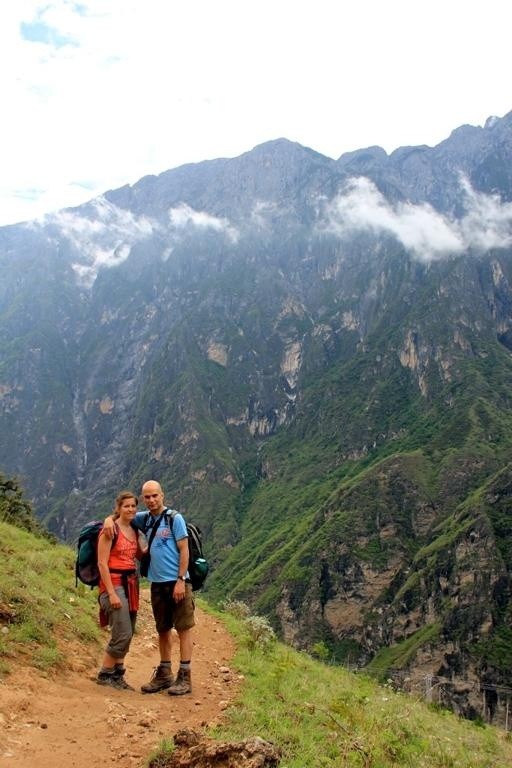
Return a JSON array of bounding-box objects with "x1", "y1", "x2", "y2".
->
[
  {"x1": 98, "y1": 480, "x2": 195, "y2": 695},
  {"x1": 97, "y1": 492, "x2": 145, "y2": 690}
]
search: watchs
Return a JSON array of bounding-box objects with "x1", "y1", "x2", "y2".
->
[{"x1": 178, "y1": 575, "x2": 186, "y2": 581}]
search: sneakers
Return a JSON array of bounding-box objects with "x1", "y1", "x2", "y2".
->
[{"x1": 96, "y1": 667, "x2": 135, "y2": 694}]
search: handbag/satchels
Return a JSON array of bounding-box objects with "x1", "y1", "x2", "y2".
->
[{"x1": 140, "y1": 551, "x2": 152, "y2": 578}]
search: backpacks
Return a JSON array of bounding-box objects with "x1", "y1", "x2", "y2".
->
[
  {"x1": 183, "y1": 522, "x2": 210, "y2": 596},
  {"x1": 73, "y1": 518, "x2": 105, "y2": 591}
]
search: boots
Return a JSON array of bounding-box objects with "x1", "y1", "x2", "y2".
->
[
  {"x1": 140, "y1": 665, "x2": 174, "y2": 694},
  {"x1": 166, "y1": 667, "x2": 193, "y2": 696}
]
